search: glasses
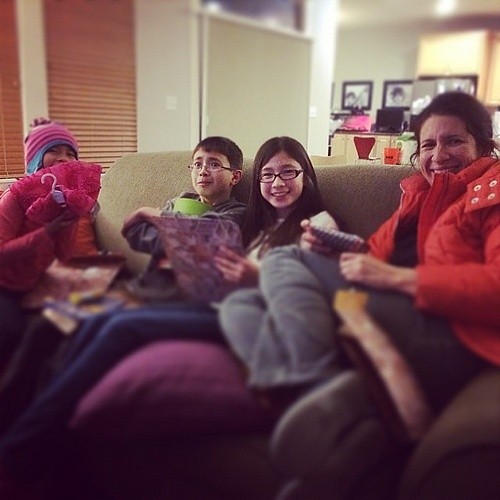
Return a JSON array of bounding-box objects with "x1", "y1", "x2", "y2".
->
[
  {"x1": 188, "y1": 162, "x2": 238, "y2": 174},
  {"x1": 257, "y1": 169, "x2": 304, "y2": 183}
]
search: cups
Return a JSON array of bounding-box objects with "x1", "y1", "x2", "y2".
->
[
  {"x1": 395, "y1": 138, "x2": 418, "y2": 166},
  {"x1": 173, "y1": 198, "x2": 214, "y2": 217},
  {"x1": 384, "y1": 147, "x2": 399, "y2": 165}
]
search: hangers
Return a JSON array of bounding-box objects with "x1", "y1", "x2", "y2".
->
[{"x1": 25, "y1": 173, "x2": 95, "y2": 216}]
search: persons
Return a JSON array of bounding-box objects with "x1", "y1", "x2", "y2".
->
[
  {"x1": 1, "y1": 92, "x2": 499, "y2": 500},
  {"x1": 1, "y1": 117, "x2": 98, "y2": 371}
]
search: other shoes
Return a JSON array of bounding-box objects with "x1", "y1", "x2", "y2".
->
[
  {"x1": 275, "y1": 420, "x2": 389, "y2": 500},
  {"x1": 272, "y1": 371, "x2": 370, "y2": 470}
]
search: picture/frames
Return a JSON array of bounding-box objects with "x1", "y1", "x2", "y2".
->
[{"x1": 341, "y1": 81, "x2": 411, "y2": 110}]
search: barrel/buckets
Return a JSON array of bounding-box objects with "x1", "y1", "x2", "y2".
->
[{"x1": 376, "y1": 109, "x2": 403, "y2": 133}]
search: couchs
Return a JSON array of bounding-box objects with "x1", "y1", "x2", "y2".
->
[{"x1": 95, "y1": 152, "x2": 419, "y2": 257}]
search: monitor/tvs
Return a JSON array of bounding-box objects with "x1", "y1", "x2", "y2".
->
[{"x1": 376, "y1": 110, "x2": 404, "y2": 131}]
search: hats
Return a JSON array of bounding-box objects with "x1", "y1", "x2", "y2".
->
[{"x1": 24, "y1": 117, "x2": 79, "y2": 175}]
